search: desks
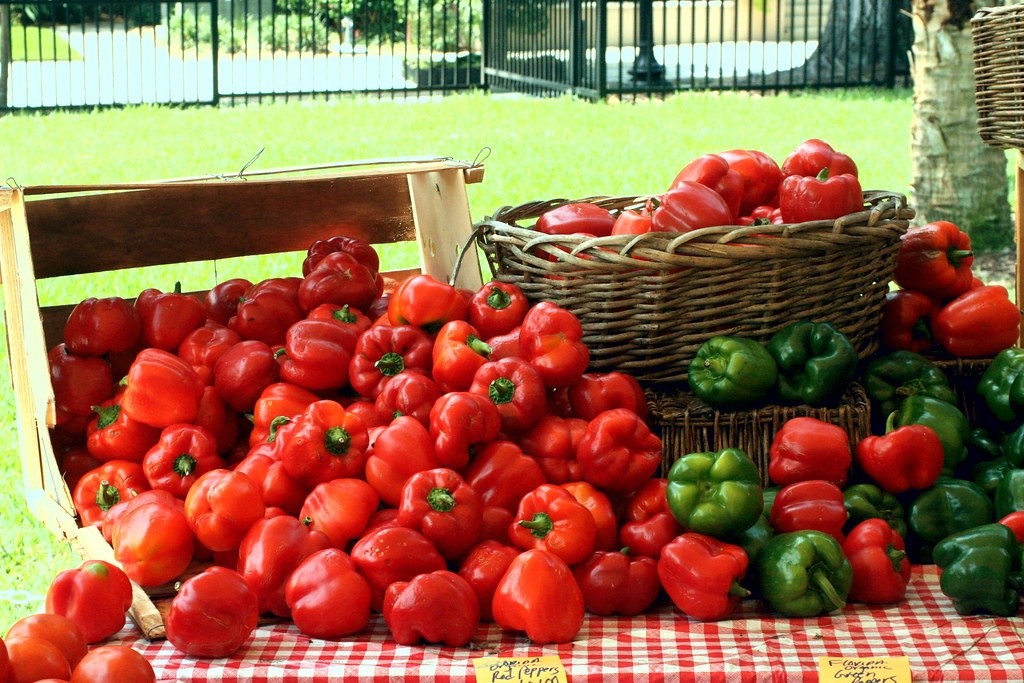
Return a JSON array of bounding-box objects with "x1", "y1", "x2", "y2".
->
[{"x1": 113, "y1": 562, "x2": 1024, "y2": 683}]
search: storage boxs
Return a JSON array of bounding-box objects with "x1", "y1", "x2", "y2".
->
[{"x1": 0, "y1": 157, "x2": 486, "y2": 642}]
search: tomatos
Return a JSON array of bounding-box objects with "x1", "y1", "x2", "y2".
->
[{"x1": 0, "y1": 613, "x2": 157, "y2": 683}]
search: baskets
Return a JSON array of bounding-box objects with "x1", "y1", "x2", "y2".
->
[
  {"x1": 473, "y1": 189, "x2": 915, "y2": 387},
  {"x1": 971, "y1": 4, "x2": 1024, "y2": 150},
  {"x1": 922, "y1": 359, "x2": 1002, "y2": 430},
  {"x1": 643, "y1": 386, "x2": 873, "y2": 492}
]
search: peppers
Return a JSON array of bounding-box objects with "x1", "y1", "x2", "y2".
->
[
  {"x1": 534, "y1": 139, "x2": 863, "y2": 276},
  {"x1": 44, "y1": 222, "x2": 1024, "y2": 655}
]
